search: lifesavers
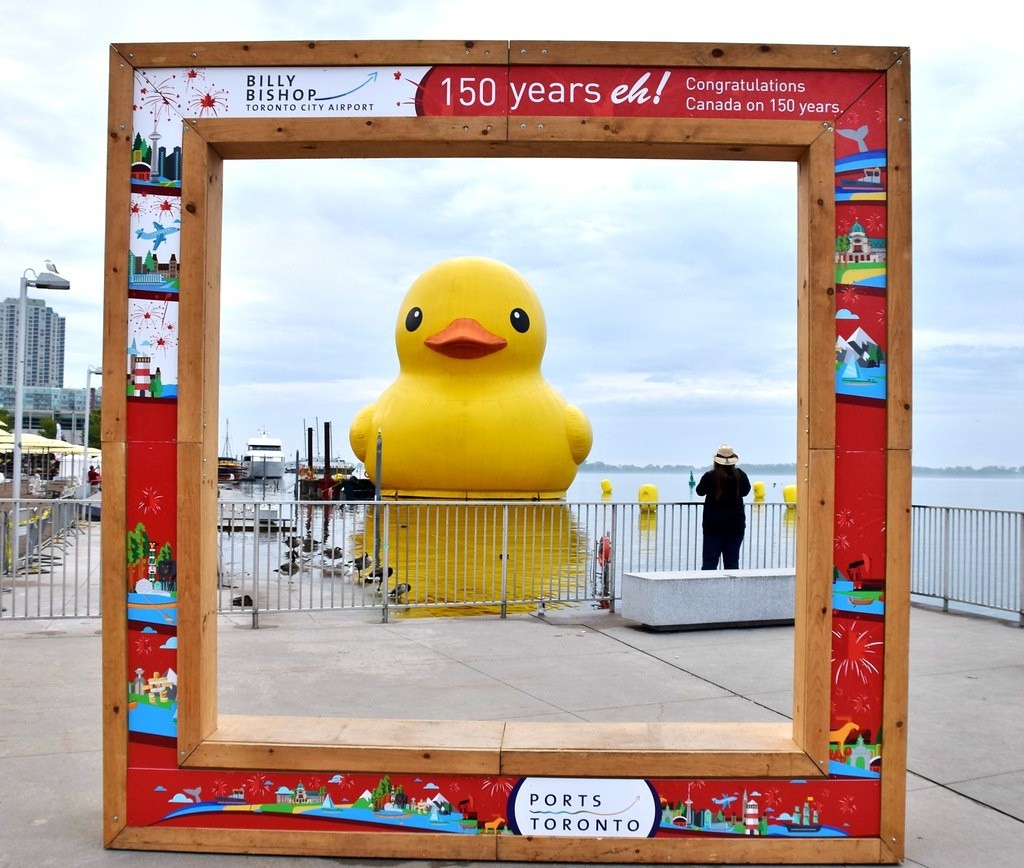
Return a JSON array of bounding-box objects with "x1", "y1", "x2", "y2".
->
[
  {"x1": 364, "y1": 471, "x2": 369, "y2": 477},
  {"x1": 598, "y1": 536, "x2": 609, "y2": 566},
  {"x1": 299, "y1": 468, "x2": 303, "y2": 476}
]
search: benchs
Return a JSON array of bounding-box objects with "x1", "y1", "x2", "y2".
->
[
  {"x1": 619, "y1": 567, "x2": 800, "y2": 628},
  {"x1": 43, "y1": 480, "x2": 68, "y2": 497}
]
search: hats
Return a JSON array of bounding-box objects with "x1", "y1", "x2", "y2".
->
[{"x1": 714, "y1": 446, "x2": 738, "y2": 465}]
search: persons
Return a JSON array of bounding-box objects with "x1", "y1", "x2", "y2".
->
[
  {"x1": 88, "y1": 465, "x2": 102, "y2": 491},
  {"x1": 695, "y1": 445, "x2": 751, "y2": 570}
]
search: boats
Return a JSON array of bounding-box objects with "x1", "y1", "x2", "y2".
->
[
  {"x1": 80, "y1": 490, "x2": 101, "y2": 518},
  {"x1": 286, "y1": 417, "x2": 378, "y2": 500},
  {"x1": 218, "y1": 419, "x2": 249, "y2": 485},
  {"x1": 242, "y1": 424, "x2": 284, "y2": 480}
]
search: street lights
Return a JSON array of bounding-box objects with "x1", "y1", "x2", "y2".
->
[
  {"x1": 81, "y1": 365, "x2": 104, "y2": 518},
  {"x1": 12, "y1": 262, "x2": 72, "y2": 576}
]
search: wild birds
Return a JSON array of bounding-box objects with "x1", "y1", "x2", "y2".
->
[
  {"x1": 283, "y1": 536, "x2": 323, "y2": 559},
  {"x1": 273, "y1": 562, "x2": 299, "y2": 583},
  {"x1": 318, "y1": 547, "x2": 343, "y2": 560},
  {"x1": 348, "y1": 552, "x2": 373, "y2": 578},
  {"x1": 387, "y1": 583, "x2": 411, "y2": 598},
  {"x1": 365, "y1": 567, "x2": 393, "y2": 591},
  {"x1": 233, "y1": 595, "x2": 253, "y2": 606}
]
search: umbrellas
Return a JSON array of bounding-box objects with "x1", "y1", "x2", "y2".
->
[{"x1": 0, "y1": 419, "x2": 102, "y2": 499}]
